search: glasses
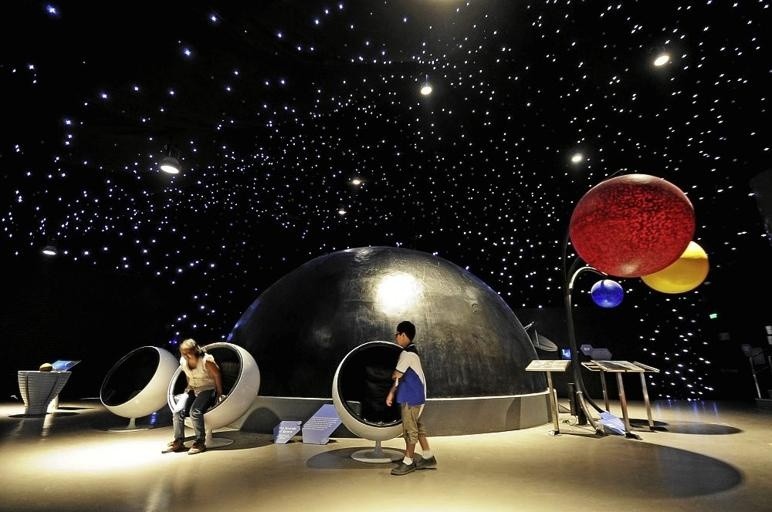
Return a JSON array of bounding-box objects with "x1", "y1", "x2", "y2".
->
[{"x1": 394, "y1": 332, "x2": 402, "y2": 338}]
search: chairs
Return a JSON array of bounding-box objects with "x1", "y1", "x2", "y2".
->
[
  {"x1": 99, "y1": 345, "x2": 179, "y2": 434},
  {"x1": 331, "y1": 340, "x2": 426, "y2": 464},
  {"x1": 165, "y1": 341, "x2": 261, "y2": 449}
]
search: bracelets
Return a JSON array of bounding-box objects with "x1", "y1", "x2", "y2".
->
[{"x1": 216, "y1": 391, "x2": 222, "y2": 394}]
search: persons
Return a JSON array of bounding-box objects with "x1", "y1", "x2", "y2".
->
[
  {"x1": 385, "y1": 320, "x2": 438, "y2": 475},
  {"x1": 161, "y1": 338, "x2": 224, "y2": 454}
]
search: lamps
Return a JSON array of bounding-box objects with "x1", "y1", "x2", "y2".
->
[
  {"x1": 157, "y1": 141, "x2": 183, "y2": 176},
  {"x1": 37, "y1": 225, "x2": 62, "y2": 256},
  {"x1": 648, "y1": 45, "x2": 670, "y2": 67}
]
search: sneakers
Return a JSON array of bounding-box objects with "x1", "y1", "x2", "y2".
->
[
  {"x1": 415, "y1": 456, "x2": 438, "y2": 470},
  {"x1": 187, "y1": 442, "x2": 206, "y2": 455},
  {"x1": 391, "y1": 461, "x2": 416, "y2": 475},
  {"x1": 161, "y1": 442, "x2": 185, "y2": 453}
]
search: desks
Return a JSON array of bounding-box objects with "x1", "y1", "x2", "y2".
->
[{"x1": 17, "y1": 369, "x2": 72, "y2": 415}]
